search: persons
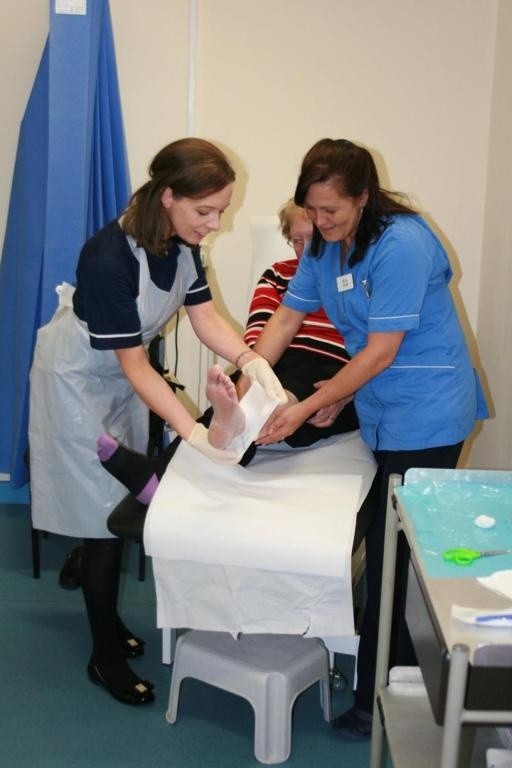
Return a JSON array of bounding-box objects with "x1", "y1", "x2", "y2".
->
[
  {"x1": 97, "y1": 194, "x2": 363, "y2": 507},
  {"x1": 233, "y1": 137, "x2": 494, "y2": 743},
  {"x1": 24, "y1": 135, "x2": 292, "y2": 708}
]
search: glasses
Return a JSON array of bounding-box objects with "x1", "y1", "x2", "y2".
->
[{"x1": 287, "y1": 235, "x2": 314, "y2": 248}]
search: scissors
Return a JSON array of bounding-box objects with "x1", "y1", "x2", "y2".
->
[{"x1": 442, "y1": 548, "x2": 511, "y2": 564}]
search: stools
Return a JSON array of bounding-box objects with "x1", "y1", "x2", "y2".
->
[{"x1": 161, "y1": 628, "x2": 331, "y2": 766}]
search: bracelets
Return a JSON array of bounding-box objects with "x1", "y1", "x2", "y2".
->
[{"x1": 233, "y1": 347, "x2": 254, "y2": 364}]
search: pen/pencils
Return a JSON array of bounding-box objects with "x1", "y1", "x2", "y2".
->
[{"x1": 478, "y1": 615, "x2": 512, "y2": 621}]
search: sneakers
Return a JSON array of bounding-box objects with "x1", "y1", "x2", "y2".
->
[{"x1": 331, "y1": 700, "x2": 372, "y2": 742}]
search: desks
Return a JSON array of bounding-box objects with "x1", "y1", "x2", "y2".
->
[{"x1": 368, "y1": 467, "x2": 512, "y2": 767}]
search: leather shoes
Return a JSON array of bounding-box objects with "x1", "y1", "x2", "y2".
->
[
  {"x1": 119, "y1": 632, "x2": 145, "y2": 657},
  {"x1": 57, "y1": 544, "x2": 85, "y2": 590},
  {"x1": 87, "y1": 654, "x2": 155, "y2": 705}
]
